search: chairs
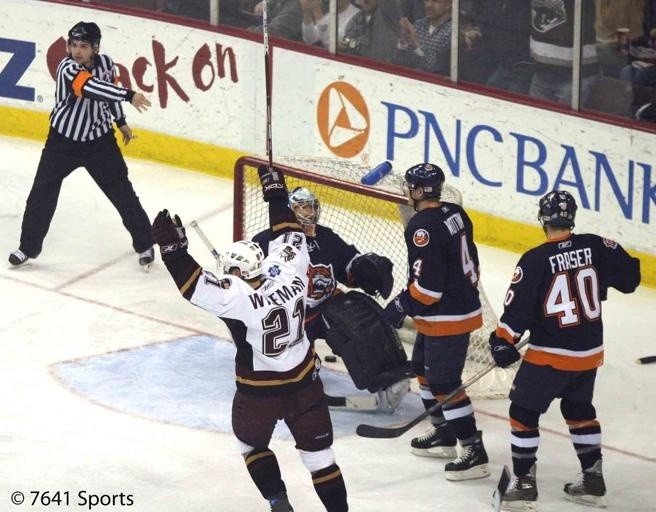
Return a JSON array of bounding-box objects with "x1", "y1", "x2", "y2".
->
[{"x1": 505, "y1": 59, "x2": 656, "y2": 128}]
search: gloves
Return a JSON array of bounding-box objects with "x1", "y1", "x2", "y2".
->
[
  {"x1": 259, "y1": 163, "x2": 289, "y2": 202},
  {"x1": 488, "y1": 331, "x2": 522, "y2": 369},
  {"x1": 380, "y1": 289, "x2": 415, "y2": 329},
  {"x1": 152, "y1": 208, "x2": 187, "y2": 261},
  {"x1": 350, "y1": 252, "x2": 394, "y2": 300}
]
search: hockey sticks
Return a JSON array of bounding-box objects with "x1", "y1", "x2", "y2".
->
[
  {"x1": 356, "y1": 337, "x2": 529, "y2": 438},
  {"x1": 190, "y1": 220, "x2": 377, "y2": 411}
]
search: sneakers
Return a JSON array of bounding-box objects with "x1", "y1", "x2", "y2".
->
[
  {"x1": 373, "y1": 378, "x2": 409, "y2": 414},
  {"x1": 8, "y1": 247, "x2": 30, "y2": 266},
  {"x1": 139, "y1": 246, "x2": 155, "y2": 265}
]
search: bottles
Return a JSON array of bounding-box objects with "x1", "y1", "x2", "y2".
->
[{"x1": 361, "y1": 159, "x2": 394, "y2": 187}]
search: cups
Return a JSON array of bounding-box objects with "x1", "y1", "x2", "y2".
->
[{"x1": 615, "y1": 25, "x2": 632, "y2": 57}]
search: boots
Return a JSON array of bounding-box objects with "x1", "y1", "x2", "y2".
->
[
  {"x1": 410, "y1": 423, "x2": 458, "y2": 448},
  {"x1": 564, "y1": 471, "x2": 607, "y2": 496},
  {"x1": 502, "y1": 475, "x2": 538, "y2": 501},
  {"x1": 445, "y1": 430, "x2": 489, "y2": 471}
]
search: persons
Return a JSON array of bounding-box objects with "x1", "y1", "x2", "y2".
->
[
  {"x1": 152, "y1": 162, "x2": 356, "y2": 511},
  {"x1": 489, "y1": 191, "x2": 641, "y2": 509},
  {"x1": 112, "y1": 2, "x2": 655, "y2": 123},
  {"x1": 383, "y1": 164, "x2": 494, "y2": 482},
  {"x1": 250, "y1": 182, "x2": 417, "y2": 416},
  {"x1": 8, "y1": 21, "x2": 156, "y2": 271}
]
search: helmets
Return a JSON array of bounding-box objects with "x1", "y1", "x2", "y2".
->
[
  {"x1": 67, "y1": 22, "x2": 101, "y2": 55},
  {"x1": 287, "y1": 186, "x2": 322, "y2": 238},
  {"x1": 537, "y1": 189, "x2": 577, "y2": 230},
  {"x1": 219, "y1": 239, "x2": 265, "y2": 282},
  {"x1": 401, "y1": 162, "x2": 444, "y2": 201}
]
